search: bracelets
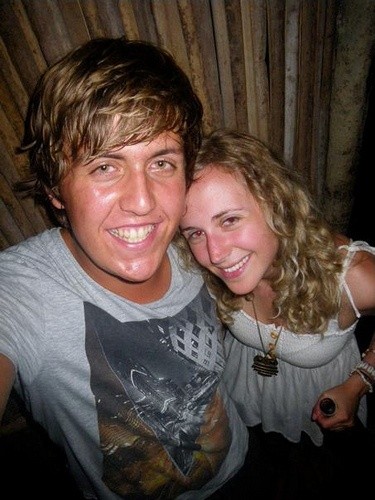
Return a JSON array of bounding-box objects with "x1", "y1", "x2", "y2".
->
[{"x1": 349, "y1": 348, "x2": 375, "y2": 394}]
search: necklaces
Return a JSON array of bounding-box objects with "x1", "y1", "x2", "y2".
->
[{"x1": 251, "y1": 292, "x2": 285, "y2": 377}]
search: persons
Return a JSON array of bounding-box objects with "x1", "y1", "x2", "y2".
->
[{"x1": 0, "y1": 37, "x2": 375, "y2": 500}]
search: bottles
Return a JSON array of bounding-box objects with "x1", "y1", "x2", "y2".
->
[{"x1": 319, "y1": 398, "x2": 372, "y2": 471}]
